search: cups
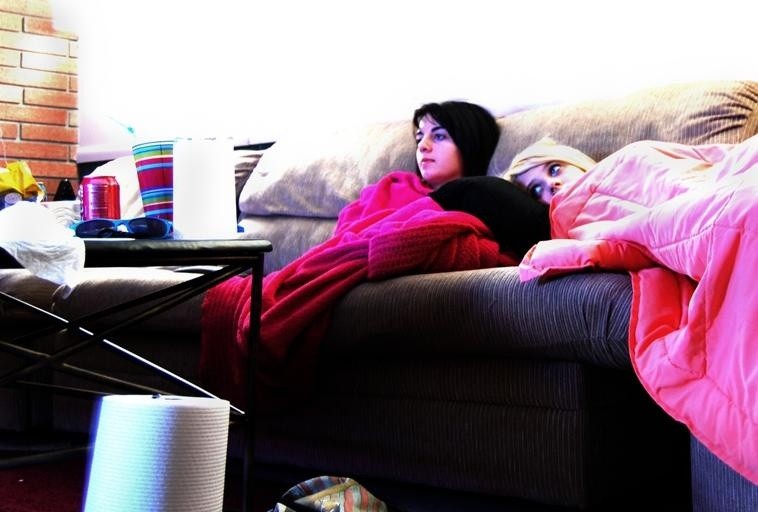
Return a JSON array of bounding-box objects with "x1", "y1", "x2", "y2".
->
[{"x1": 131, "y1": 141, "x2": 174, "y2": 240}]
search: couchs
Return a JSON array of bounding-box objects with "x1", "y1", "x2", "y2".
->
[{"x1": 1, "y1": 75, "x2": 756, "y2": 512}]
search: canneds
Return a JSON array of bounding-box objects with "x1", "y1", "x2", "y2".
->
[{"x1": 80, "y1": 175, "x2": 121, "y2": 221}]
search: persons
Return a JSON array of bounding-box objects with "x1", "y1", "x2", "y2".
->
[
  {"x1": 498, "y1": 133, "x2": 598, "y2": 202},
  {"x1": 408, "y1": 93, "x2": 501, "y2": 191}
]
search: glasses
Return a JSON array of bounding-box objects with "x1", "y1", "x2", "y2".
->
[{"x1": 68, "y1": 216, "x2": 174, "y2": 241}]
